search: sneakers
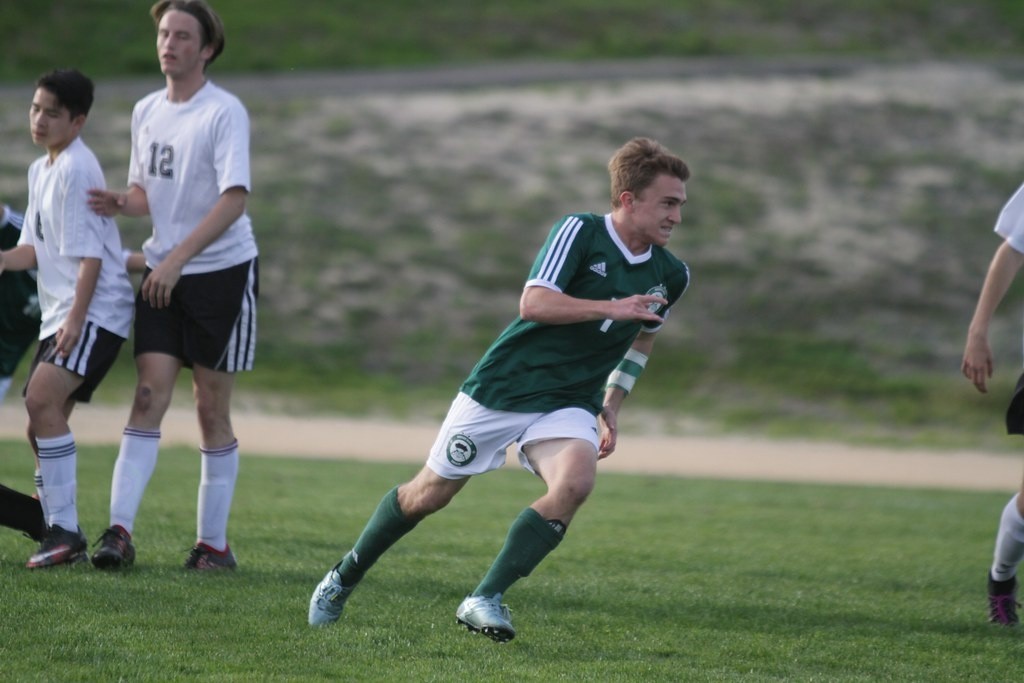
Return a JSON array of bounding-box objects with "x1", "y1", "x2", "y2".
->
[
  {"x1": 307, "y1": 559, "x2": 359, "y2": 625},
  {"x1": 91, "y1": 525, "x2": 135, "y2": 566},
  {"x1": 455, "y1": 590, "x2": 515, "y2": 643},
  {"x1": 24, "y1": 523, "x2": 88, "y2": 570},
  {"x1": 183, "y1": 542, "x2": 237, "y2": 570},
  {"x1": 987, "y1": 580, "x2": 1020, "y2": 626}
]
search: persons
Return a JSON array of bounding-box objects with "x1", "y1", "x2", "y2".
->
[
  {"x1": 961, "y1": 184, "x2": 1024, "y2": 626},
  {"x1": 1, "y1": 67, "x2": 145, "y2": 571},
  {"x1": 86, "y1": 0, "x2": 260, "y2": 571},
  {"x1": 309, "y1": 138, "x2": 690, "y2": 643}
]
